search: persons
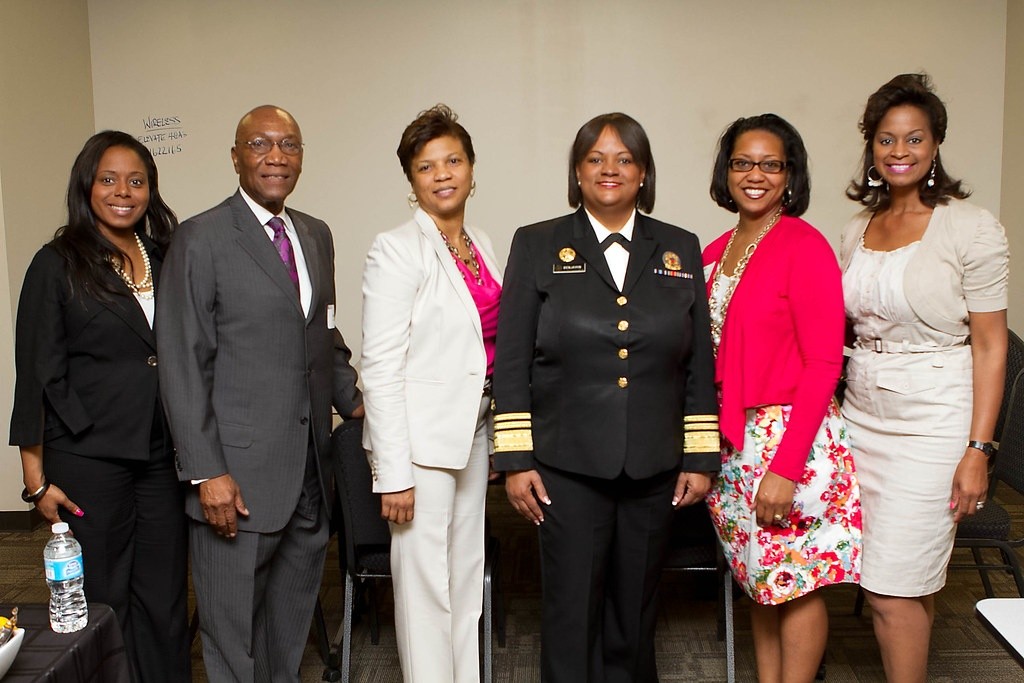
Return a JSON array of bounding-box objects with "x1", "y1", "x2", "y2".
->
[
  {"x1": 701, "y1": 113, "x2": 862, "y2": 683},
  {"x1": 9, "y1": 130, "x2": 192, "y2": 683},
  {"x1": 362, "y1": 103, "x2": 506, "y2": 683},
  {"x1": 490, "y1": 112, "x2": 723, "y2": 683},
  {"x1": 839, "y1": 69, "x2": 1011, "y2": 683},
  {"x1": 157, "y1": 103, "x2": 366, "y2": 683}
]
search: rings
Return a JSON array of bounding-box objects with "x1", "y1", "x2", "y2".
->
[
  {"x1": 775, "y1": 514, "x2": 781, "y2": 520},
  {"x1": 976, "y1": 501, "x2": 985, "y2": 510}
]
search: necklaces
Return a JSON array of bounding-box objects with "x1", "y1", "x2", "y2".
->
[
  {"x1": 105, "y1": 231, "x2": 173, "y2": 300},
  {"x1": 433, "y1": 227, "x2": 485, "y2": 287},
  {"x1": 709, "y1": 206, "x2": 783, "y2": 357}
]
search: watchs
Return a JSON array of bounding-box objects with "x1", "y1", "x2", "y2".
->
[{"x1": 967, "y1": 441, "x2": 996, "y2": 457}]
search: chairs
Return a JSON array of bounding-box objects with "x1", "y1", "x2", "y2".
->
[
  {"x1": 652, "y1": 505, "x2": 742, "y2": 683},
  {"x1": 331, "y1": 415, "x2": 507, "y2": 683},
  {"x1": 854, "y1": 332, "x2": 1024, "y2": 617}
]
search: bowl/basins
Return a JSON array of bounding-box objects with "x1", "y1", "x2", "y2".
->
[{"x1": 0, "y1": 628, "x2": 26, "y2": 679}]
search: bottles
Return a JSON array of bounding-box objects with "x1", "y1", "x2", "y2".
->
[{"x1": 43, "y1": 522, "x2": 89, "y2": 634}]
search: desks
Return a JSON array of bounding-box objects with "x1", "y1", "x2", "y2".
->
[
  {"x1": 976, "y1": 594, "x2": 1024, "y2": 669},
  {"x1": 0, "y1": 600, "x2": 132, "y2": 683}
]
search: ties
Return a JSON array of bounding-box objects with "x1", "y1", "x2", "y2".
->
[{"x1": 267, "y1": 217, "x2": 301, "y2": 304}]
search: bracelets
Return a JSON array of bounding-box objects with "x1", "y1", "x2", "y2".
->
[{"x1": 21, "y1": 479, "x2": 52, "y2": 502}]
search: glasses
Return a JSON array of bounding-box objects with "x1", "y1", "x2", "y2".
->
[
  {"x1": 727, "y1": 159, "x2": 795, "y2": 174},
  {"x1": 234, "y1": 137, "x2": 304, "y2": 156}
]
